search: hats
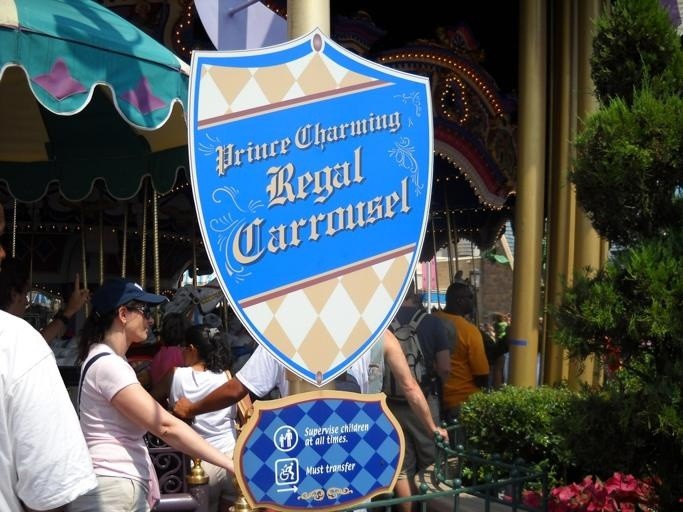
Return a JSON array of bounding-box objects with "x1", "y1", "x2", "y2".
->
[{"x1": 94, "y1": 276, "x2": 168, "y2": 316}]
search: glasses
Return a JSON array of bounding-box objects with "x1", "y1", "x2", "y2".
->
[{"x1": 130, "y1": 306, "x2": 152, "y2": 320}]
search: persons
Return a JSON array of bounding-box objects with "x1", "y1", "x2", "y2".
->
[{"x1": 0, "y1": 237, "x2": 544, "y2": 510}]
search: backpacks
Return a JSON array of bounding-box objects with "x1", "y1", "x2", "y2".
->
[{"x1": 383, "y1": 308, "x2": 429, "y2": 404}]
